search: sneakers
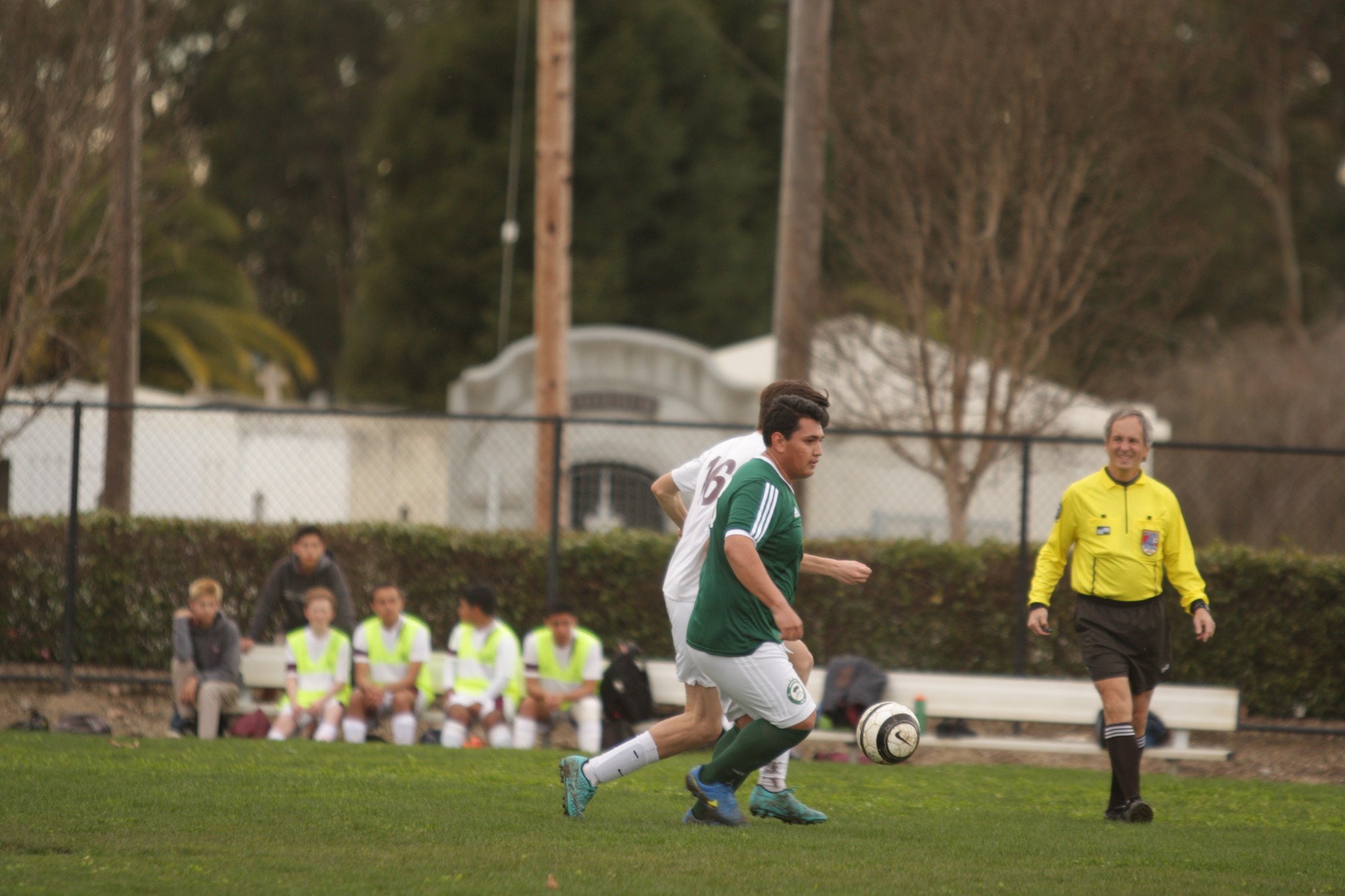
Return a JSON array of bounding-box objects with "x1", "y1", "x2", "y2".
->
[
  {"x1": 559, "y1": 755, "x2": 597, "y2": 820},
  {"x1": 685, "y1": 764, "x2": 750, "y2": 825},
  {"x1": 683, "y1": 810, "x2": 720, "y2": 827},
  {"x1": 749, "y1": 781, "x2": 828, "y2": 824}
]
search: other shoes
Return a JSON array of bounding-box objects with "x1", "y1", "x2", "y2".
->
[{"x1": 1103, "y1": 802, "x2": 1153, "y2": 822}]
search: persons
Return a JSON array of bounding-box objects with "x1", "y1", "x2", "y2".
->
[
  {"x1": 682, "y1": 392, "x2": 830, "y2": 823},
  {"x1": 1026, "y1": 406, "x2": 1218, "y2": 825},
  {"x1": 556, "y1": 377, "x2": 874, "y2": 826},
  {"x1": 506, "y1": 600, "x2": 605, "y2": 753},
  {"x1": 340, "y1": 581, "x2": 432, "y2": 745},
  {"x1": 240, "y1": 526, "x2": 355, "y2": 635},
  {"x1": 434, "y1": 583, "x2": 526, "y2": 749},
  {"x1": 167, "y1": 578, "x2": 245, "y2": 743},
  {"x1": 268, "y1": 586, "x2": 353, "y2": 744}
]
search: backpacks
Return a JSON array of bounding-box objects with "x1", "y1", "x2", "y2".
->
[{"x1": 600, "y1": 651, "x2": 653, "y2": 722}]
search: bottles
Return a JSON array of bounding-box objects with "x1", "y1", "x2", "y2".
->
[{"x1": 915, "y1": 694, "x2": 925, "y2": 734}]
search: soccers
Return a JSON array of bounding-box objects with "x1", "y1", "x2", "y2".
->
[{"x1": 857, "y1": 702, "x2": 921, "y2": 764}]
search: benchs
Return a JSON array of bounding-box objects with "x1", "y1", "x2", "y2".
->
[{"x1": 219, "y1": 644, "x2": 1241, "y2": 765}]
